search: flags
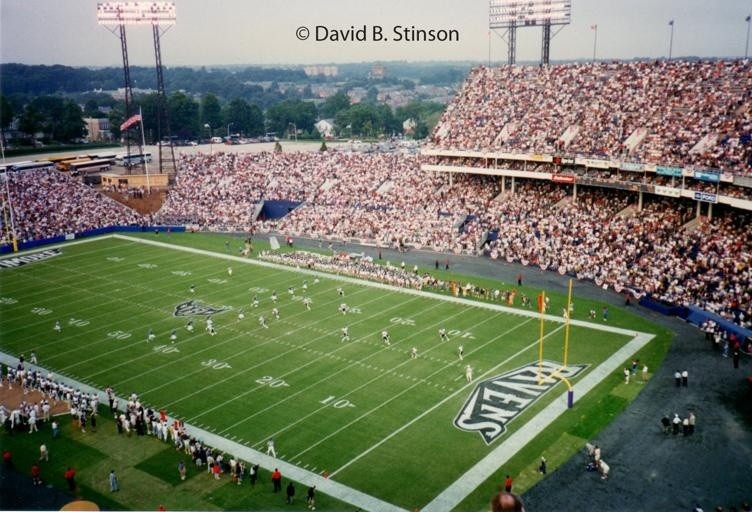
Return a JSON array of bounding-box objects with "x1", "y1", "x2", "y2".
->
[{"x1": 116, "y1": 107, "x2": 144, "y2": 133}]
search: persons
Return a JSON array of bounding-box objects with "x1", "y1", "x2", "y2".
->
[
  {"x1": 150, "y1": 132, "x2": 424, "y2": 363},
  {"x1": 597, "y1": 459, "x2": 609, "y2": 481},
  {"x1": 53, "y1": 320, "x2": 62, "y2": 333},
  {"x1": 147, "y1": 331, "x2": 157, "y2": 344},
  {"x1": 422, "y1": 55, "x2": 752, "y2": 389},
  {"x1": 532, "y1": 455, "x2": 546, "y2": 473},
  {"x1": 0, "y1": 349, "x2": 318, "y2": 510},
  {"x1": 594, "y1": 445, "x2": 599, "y2": 467},
  {"x1": 491, "y1": 489, "x2": 526, "y2": 512},
  {"x1": 0, "y1": 158, "x2": 152, "y2": 254},
  {"x1": 584, "y1": 442, "x2": 594, "y2": 462},
  {"x1": 505, "y1": 475, "x2": 512, "y2": 491},
  {"x1": 672, "y1": 413, "x2": 682, "y2": 434},
  {"x1": 682, "y1": 416, "x2": 689, "y2": 437},
  {"x1": 688, "y1": 411, "x2": 696, "y2": 435},
  {"x1": 661, "y1": 414, "x2": 670, "y2": 435}
]
